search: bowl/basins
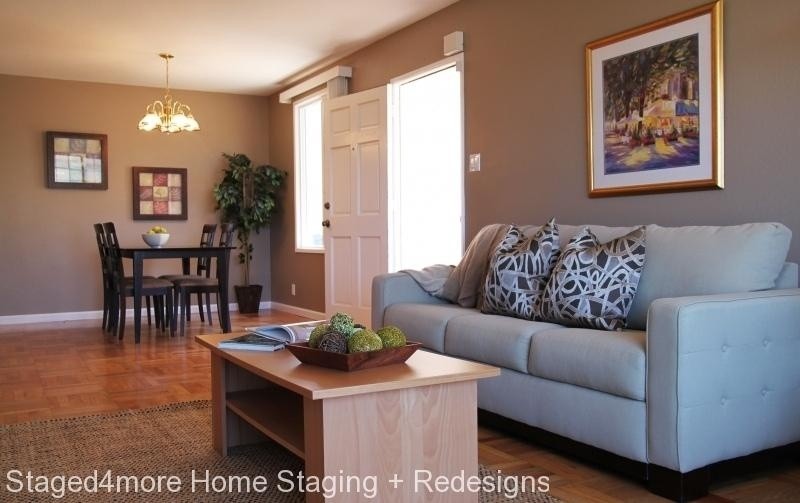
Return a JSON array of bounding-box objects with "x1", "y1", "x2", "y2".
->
[{"x1": 142, "y1": 233, "x2": 170, "y2": 248}]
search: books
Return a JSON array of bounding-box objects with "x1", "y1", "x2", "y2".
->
[
  {"x1": 246, "y1": 318, "x2": 330, "y2": 343},
  {"x1": 220, "y1": 333, "x2": 286, "y2": 352}
]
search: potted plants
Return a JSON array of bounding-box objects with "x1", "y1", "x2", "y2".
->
[{"x1": 211, "y1": 154, "x2": 288, "y2": 313}]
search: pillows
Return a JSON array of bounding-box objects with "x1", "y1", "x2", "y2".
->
[
  {"x1": 480, "y1": 220, "x2": 560, "y2": 320},
  {"x1": 535, "y1": 228, "x2": 647, "y2": 331}
]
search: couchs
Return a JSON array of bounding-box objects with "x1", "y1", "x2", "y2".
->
[{"x1": 372, "y1": 225, "x2": 800, "y2": 501}]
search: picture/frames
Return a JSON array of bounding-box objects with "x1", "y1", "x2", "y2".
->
[
  {"x1": 44, "y1": 131, "x2": 108, "y2": 189},
  {"x1": 132, "y1": 167, "x2": 188, "y2": 221},
  {"x1": 583, "y1": 2, "x2": 726, "y2": 198}
]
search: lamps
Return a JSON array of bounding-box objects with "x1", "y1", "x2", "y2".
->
[{"x1": 138, "y1": 53, "x2": 200, "y2": 134}]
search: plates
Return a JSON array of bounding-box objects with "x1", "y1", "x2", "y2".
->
[{"x1": 285, "y1": 340, "x2": 424, "y2": 372}]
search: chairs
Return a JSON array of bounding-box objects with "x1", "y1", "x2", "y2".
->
[
  {"x1": 159, "y1": 225, "x2": 234, "y2": 336},
  {"x1": 94, "y1": 223, "x2": 176, "y2": 342}
]
